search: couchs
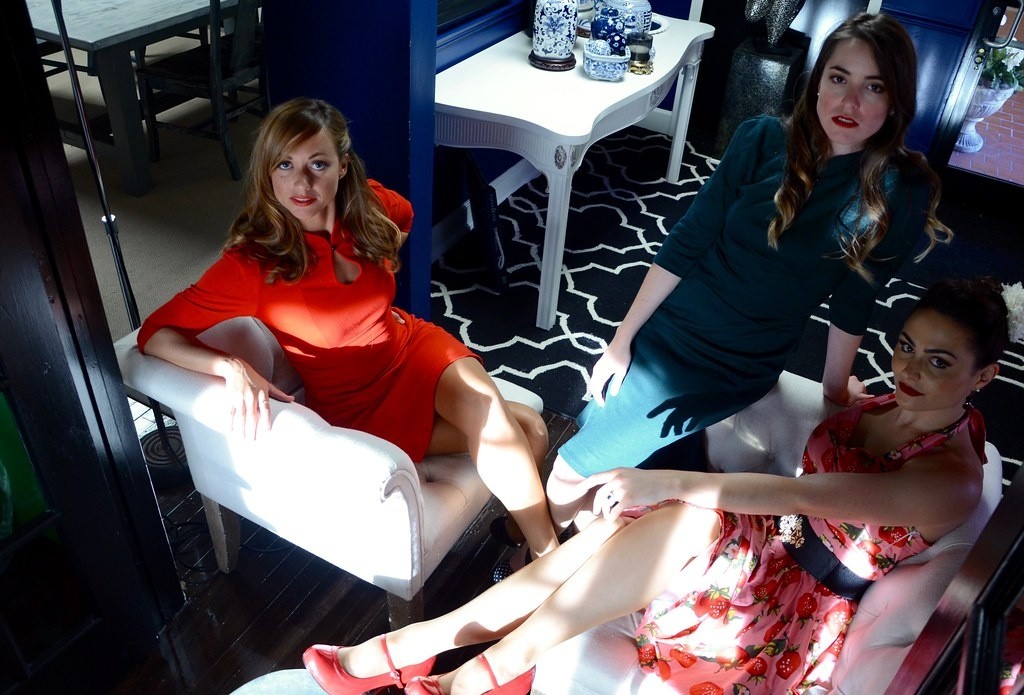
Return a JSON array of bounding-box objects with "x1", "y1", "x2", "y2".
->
[
  {"x1": 529, "y1": 368, "x2": 1004, "y2": 695},
  {"x1": 112, "y1": 243, "x2": 548, "y2": 630}
]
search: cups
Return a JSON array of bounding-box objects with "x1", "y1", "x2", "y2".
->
[{"x1": 627, "y1": 33, "x2": 654, "y2": 67}]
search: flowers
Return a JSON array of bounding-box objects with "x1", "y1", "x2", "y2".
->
[{"x1": 981, "y1": 45, "x2": 1024, "y2": 88}]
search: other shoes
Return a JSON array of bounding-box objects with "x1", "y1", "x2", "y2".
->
[{"x1": 490, "y1": 516, "x2": 522, "y2": 549}]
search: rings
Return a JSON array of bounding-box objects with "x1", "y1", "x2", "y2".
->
[
  {"x1": 606, "y1": 493, "x2": 614, "y2": 500},
  {"x1": 259, "y1": 400, "x2": 270, "y2": 404}
]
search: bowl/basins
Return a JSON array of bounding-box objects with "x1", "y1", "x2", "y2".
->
[{"x1": 582, "y1": 43, "x2": 631, "y2": 82}]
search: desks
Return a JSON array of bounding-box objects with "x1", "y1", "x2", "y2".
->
[
  {"x1": 429, "y1": 12, "x2": 720, "y2": 333},
  {"x1": 27, "y1": 0, "x2": 238, "y2": 206}
]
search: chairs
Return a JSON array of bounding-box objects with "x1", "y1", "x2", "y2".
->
[{"x1": 140, "y1": 0, "x2": 277, "y2": 185}]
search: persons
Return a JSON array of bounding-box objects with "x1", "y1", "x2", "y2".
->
[
  {"x1": 137, "y1": 96, "x2": 560, "y2": 562},
  {"x1": 490, "y1": 10, "x2": 916, "y2": 659},
  {"x1": 302, "y1": 271, "x2": 1024, "y2": 695}
]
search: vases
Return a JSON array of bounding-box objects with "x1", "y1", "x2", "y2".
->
[{"x1": 953, "y1": 85, "x2": 1015, "y2": 155}]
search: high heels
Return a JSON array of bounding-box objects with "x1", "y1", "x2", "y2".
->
[
  {"x1": 404, "y1": 653, "x2": 536, "y2": 695},
  {"x1": 492, "y1": 547, "x2": 532, "y2": 583},
  {"x1": 302, "y1": 634, "x2": 437, "y2": 695}
]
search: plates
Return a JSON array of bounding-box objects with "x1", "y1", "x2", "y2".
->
[{"x1": 577, "y1": 13, "x2": 669, "y2": 38}]
